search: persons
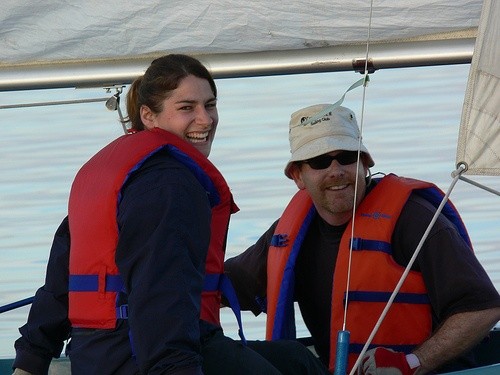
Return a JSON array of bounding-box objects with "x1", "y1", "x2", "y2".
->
[
  {"x1": 11, "y1": 54, "x2": 324, "y2": 375},
  {"x1": 221, "y1": 104, "x2": 500, "y2": 375}
]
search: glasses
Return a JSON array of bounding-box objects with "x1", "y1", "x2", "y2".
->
[{"x1": 294, "y1": 150, "x2": 367, "y2": 170}]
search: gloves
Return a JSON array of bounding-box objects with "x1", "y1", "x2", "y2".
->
[{"x1": 358, "y1": 346, "x2": 422, "y2": 375}]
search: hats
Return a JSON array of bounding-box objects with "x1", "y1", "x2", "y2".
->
[{"x1": 284, "y1": 104, "x2": 375, "y2": 179}]
